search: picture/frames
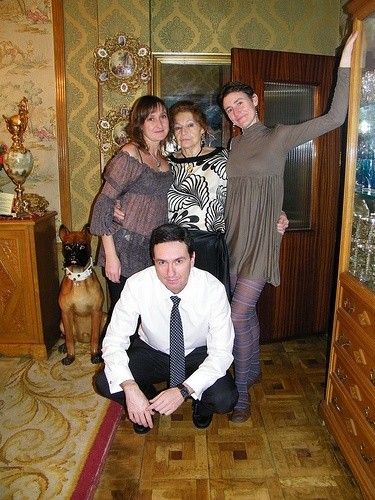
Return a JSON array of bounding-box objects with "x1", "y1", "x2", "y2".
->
[
  {"x1": 96, "y1": 30, "x2": 153, "y2": 95},
  {"x1": 96, "y1": 104, "x2": 132, "y2": 160}
]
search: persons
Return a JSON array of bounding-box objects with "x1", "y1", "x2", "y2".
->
[
  {"x1": 216, "y1": 28, "x2": 361, "y2": 422},
  {"x1": 113, "y1": 99, "x2": 289, "y2": 304},
  {"x1": 91, "y1": 222, "x2": 239, "y2": 433},
  {"x1": 89, "y1": 94, "x2": 176, "y2": 364}
]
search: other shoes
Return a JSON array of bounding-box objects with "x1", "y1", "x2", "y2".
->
[
  {"x1": 247, "y1": 370, "x2": 262, "y2": 389},
  {"x1": 231, "y1": 392, "x2": 251, "y2": 423}
]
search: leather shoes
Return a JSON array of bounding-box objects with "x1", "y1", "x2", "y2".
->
[
  {"x1": 191, "y1": 396, "x2": 213, "y2": 429},
  {"x1": 133, "y1": 402, "x2": 154, "y2": 434}
]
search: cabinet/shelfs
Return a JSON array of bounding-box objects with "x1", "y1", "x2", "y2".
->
[
  {"x1": 319, "y1": 0, "x2": 375, "y2": 500},
  {"x1": 0, "y1": 212, "x2": 63, "y2": 360}
]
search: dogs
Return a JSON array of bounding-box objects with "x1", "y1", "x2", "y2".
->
[{"x1": 58, "y1": 223, "x2": 108, "y2": 365}]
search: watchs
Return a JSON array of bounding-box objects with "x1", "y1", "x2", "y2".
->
[{"x1": 177, "y1": 383, "x2": 191, "y2": 398}]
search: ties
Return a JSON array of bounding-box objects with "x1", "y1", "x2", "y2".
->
[{"x1": 169, "y1": 296, "x2": 186, "y2": 388}]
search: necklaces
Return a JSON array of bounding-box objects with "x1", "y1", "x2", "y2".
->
[
  {"x1": 180, "y1": 146, "x2": 203, "y2": 173},
  {"x1": 148, "y1": 149, "x2": 162, "y2": 167}
]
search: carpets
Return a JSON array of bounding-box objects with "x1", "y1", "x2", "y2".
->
[{"x1": 0, "y1": 336, "x2": 125, "y2": 500}]
